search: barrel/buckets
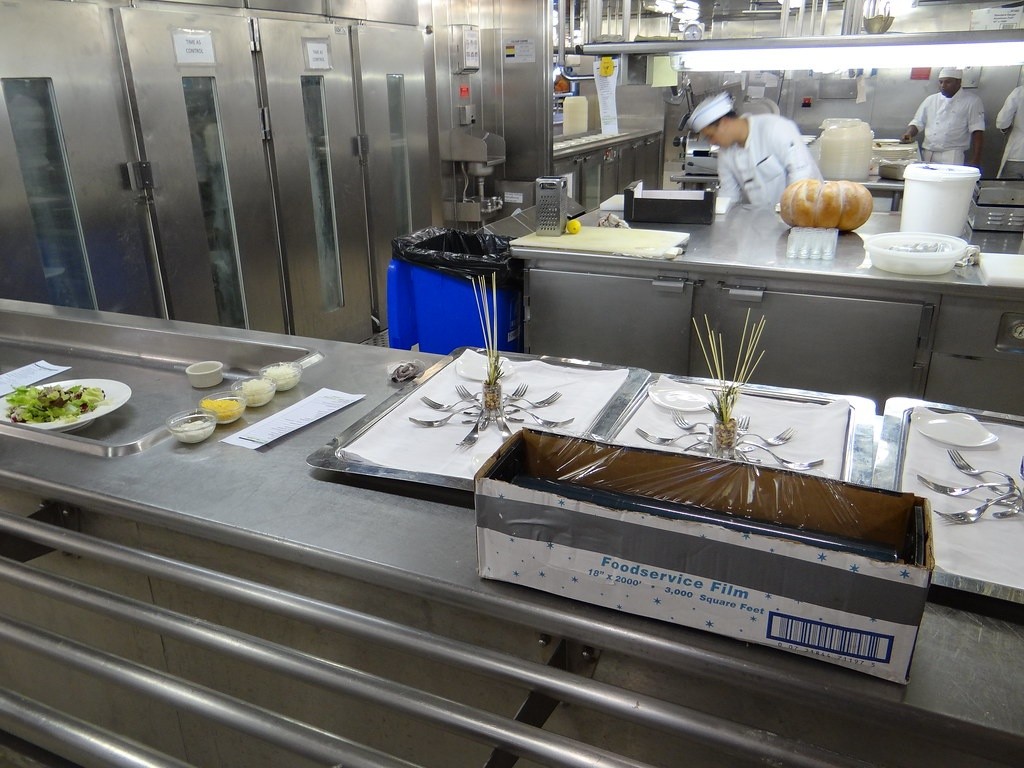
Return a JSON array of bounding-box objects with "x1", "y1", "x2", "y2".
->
[{"x1": 900, "y1": 164, "x2": 980, "y2": 236}]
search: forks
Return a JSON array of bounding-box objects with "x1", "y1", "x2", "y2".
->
[
  {"x1": 409, "y1": 383, "x2": 575, "y2": 447},
  {"x1": 636, "y1": 409, "x2": 824, "y2": 472},
  {"x1": 917, "y1": 449, "x2": 1024, "y2": 524}
]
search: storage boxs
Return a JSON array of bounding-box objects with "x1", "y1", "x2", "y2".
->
[{"x1": 467, "y1": 432, "x2": 940, "y2": 698}]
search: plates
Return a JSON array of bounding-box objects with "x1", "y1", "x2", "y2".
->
[
  {"x1": 0, "y1": 378, "x2": 132, "y2": 432},
  {"x1": 647, "y1": 383, "x2": 711, "y2": 410},
  {"x1": 912, "y1": 407, "x2": 999, "y2": 447},
  {"x1": 457, "y1": 357, "x2": 513, "y2": 380}
]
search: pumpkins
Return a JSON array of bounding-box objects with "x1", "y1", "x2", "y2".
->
[{"x1": 780, "y1": 178, "x2": 873, "y2": 233}]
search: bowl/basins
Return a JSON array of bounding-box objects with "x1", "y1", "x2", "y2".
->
[
  {"x1": 863, "y1": 232, "x2": 967, "y2": 276},
  {"x1": 863, "y1": 16, "x2": 894, "y2": 34},
  {"x1": 231, "y1": 375, "x2": 276, "y2": 407},
  {"x1": 259, "y1": 362, "x2": 303, "y2": 391},
  {"x1": 165, "y1": 408, "x2": 218, "y2": 443},
  {"x1": 199, "y1": 391, "x2": 247, "y2": 424},
  {"x1": 186, "y1": 360, "x2": 223, "y2": 388},
  {"x1": 819, "y1": 119, "x2": 872, "y2": 182}
]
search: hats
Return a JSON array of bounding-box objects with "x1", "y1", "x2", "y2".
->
[
  {"x1": 939, "y1": 67, "x2": 963, "y2": 79},
  {"x1": 686, "y1": 91, "x2": 733, "y2": 134}
]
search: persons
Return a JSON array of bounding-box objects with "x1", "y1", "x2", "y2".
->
[
  {"x1": 688, "y1": 92, "x2": 822, "y2": 208},
  {"x1": 900, "y1": 68, "x2": 986, "y2": 169},
  {"x1": 996, "y1": 85, "x2": 1024, "y2": 180}
]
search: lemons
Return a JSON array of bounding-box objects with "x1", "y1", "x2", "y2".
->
[{"x1": 566, "y1": 219, "x2": 581, "y2": 234}]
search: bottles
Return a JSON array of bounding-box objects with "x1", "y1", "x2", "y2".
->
[{"x1": 563, "y1": 96, "x2": 588, "y2": 136}]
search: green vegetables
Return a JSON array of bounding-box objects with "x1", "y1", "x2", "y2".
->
[{"x1": 5, "y1": 385, "x2": 115, "y2": 425}]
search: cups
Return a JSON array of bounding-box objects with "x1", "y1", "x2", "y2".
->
[{"x1": 787, "y1": 226, "x2": 838, "y2": 260}]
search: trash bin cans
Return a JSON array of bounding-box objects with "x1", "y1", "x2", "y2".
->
[{"x1": 385, "y1": 227, "x2": 523, "y2": 358}]
